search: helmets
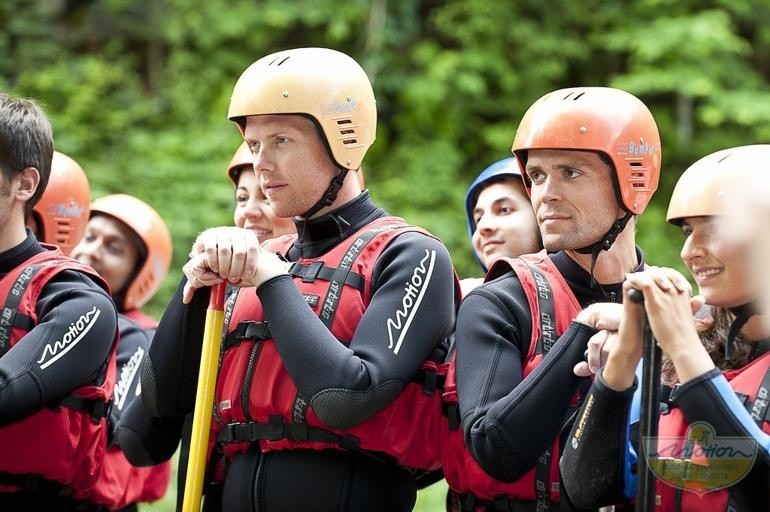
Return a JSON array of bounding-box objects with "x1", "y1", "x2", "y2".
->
[
  {"x1": 89, "y1": 191, "x2": 174, "y2": 314},
  {"x1": 228, "y1": 140, "x2": 367, "y2": 198},
  {"x1": 465, "y1": 156, "x2": 522, "y2": 274},
  {"x1": 228, "y1": 47, "x2": 378, "y2": 173},
  {"x1": 31, "y1": 148, "x2": 92, "y2": 257},
  {"x1": 512, "y1": 87, "x2": 662, "y2": 216},
  {"x1": 665, "y1": 144, "x2": 770, "y2": 227}
]
